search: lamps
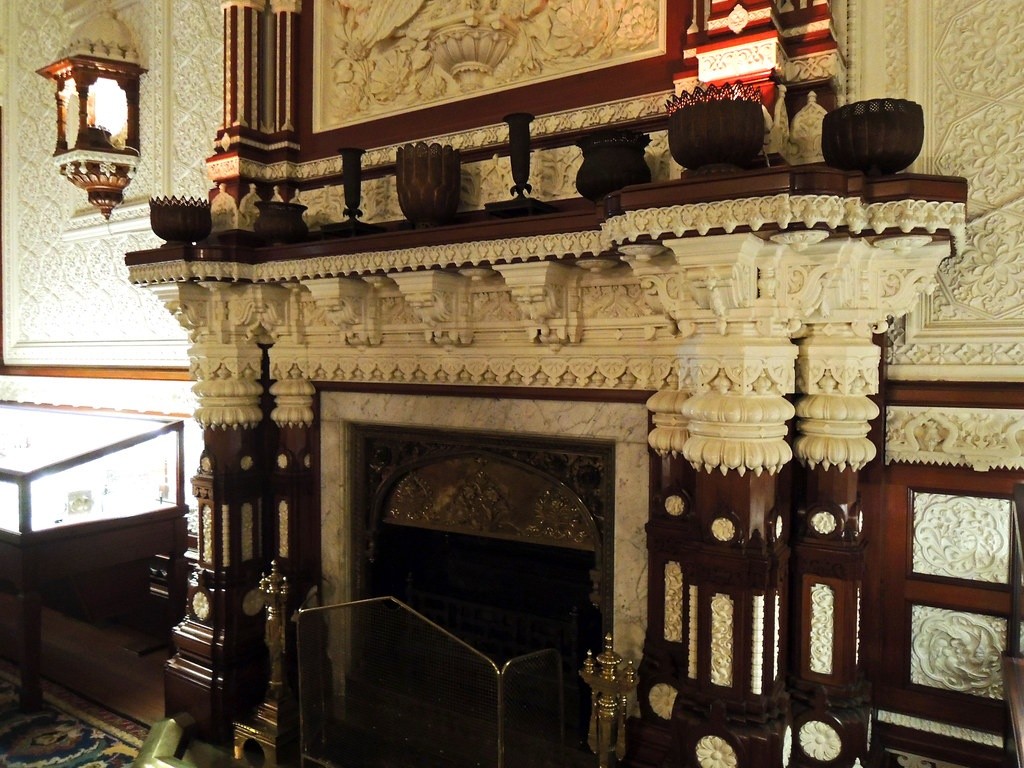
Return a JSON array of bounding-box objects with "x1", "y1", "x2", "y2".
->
[{"x1": 34, "y1": 56, "x2": 149, "y2": 223}]
